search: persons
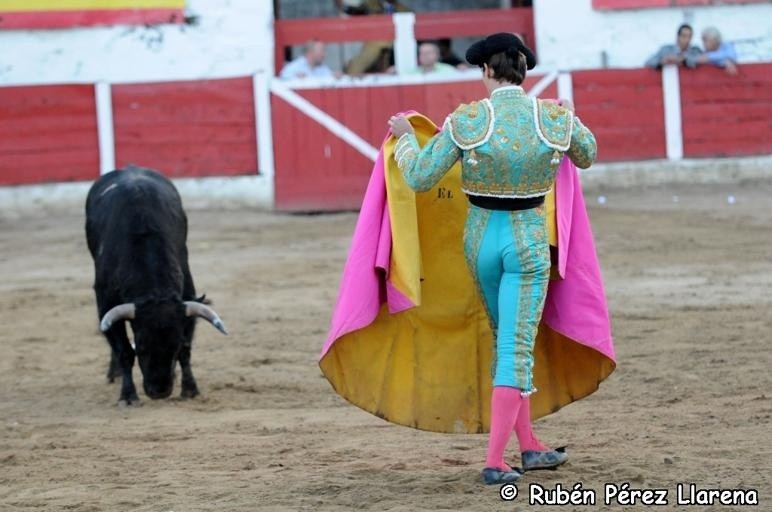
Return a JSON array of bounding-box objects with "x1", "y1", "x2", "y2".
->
[
  {"x1": 276, "y1": 0, "x2": 476, "y2": 82},
  {"x1": 691, "y1": 27, "x2": 740, "y2": 77},
  {"x1": 641, "y1": 24, "x2": 706, "y2": 69},
  {"x1": 385, "y1": 30, "x2": 600, "y2": 488}
]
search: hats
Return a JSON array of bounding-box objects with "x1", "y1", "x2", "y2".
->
[{"x1": 465, "y1": 32, "x2": 536, "y2": 70}]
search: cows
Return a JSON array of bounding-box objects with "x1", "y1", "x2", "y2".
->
[{"x1": 83, "y1": 163, "x2": 230, "y2": 405}]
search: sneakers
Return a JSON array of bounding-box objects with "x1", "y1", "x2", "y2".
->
[
  {"x1": 483, "y1": 463, "x2": 525, "y2": 484},
  {"x1": 520, "y1": 447, "x2": 570, "y2": 472}
]
